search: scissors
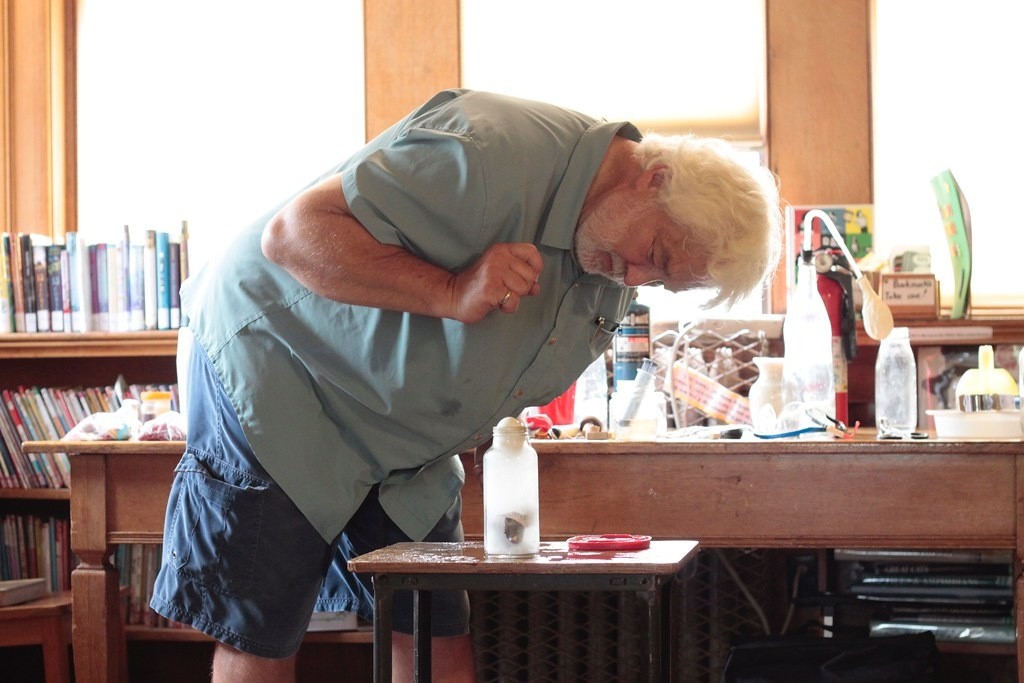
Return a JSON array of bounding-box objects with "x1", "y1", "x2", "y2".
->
[{"x1": 879, "y1": 421, "x2": 930, "y2": 441}]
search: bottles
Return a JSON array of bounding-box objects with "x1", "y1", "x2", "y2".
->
[
  {"x1": 482, "y1": 426, "x2": 541, "y2": 561},
  {"x1": 782, "y1": 255, "x2": 833, "y2": 438},
  {"x1": 750, "y1": 356, "x2": 786, "y2": 440},
  {"x1": 874, "y1": 326, "x2": 918, "y2": 435}
]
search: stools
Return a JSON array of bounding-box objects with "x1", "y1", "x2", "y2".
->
[{"x1": 0, "y1": 587, "x2": 130, "y2": 683}]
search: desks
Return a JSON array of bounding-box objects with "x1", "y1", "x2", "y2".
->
[
  {"x1": 347, "y1": 540, "x2": 701, "y2": 683},
  {"x1": 22, "y1": 440, "x2": 1024, "y2": 683}
]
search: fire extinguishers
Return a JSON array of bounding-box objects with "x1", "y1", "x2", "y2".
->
[{"x1": 813, "y1": 245, "x2": 858, "y2": 434}]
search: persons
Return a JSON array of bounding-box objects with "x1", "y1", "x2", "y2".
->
[{"x1": 149, "y1": 89, "x2": 782, "y2": 683}]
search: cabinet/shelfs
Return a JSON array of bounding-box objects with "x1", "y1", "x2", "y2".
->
[{"x1": 0, "y1": 329, "x2": 180, "y2": 644}]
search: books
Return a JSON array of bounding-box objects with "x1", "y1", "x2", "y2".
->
[
  {"x1": 0, "y1": 514, "x2": 186, "y2": 628},
  {"x1": 835, "y1": 548, "x2": 1015, "y2": 643},
  {"x1": 0, "y1": 220, "x2": 189, "y2": 333},
  {"x1": 0, "y1": 375, "x2": 179, "y2": 489}
]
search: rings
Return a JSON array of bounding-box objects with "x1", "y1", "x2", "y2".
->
[{"x1": 501, "y1": 290, "x2": 511, "y2": 305}]
